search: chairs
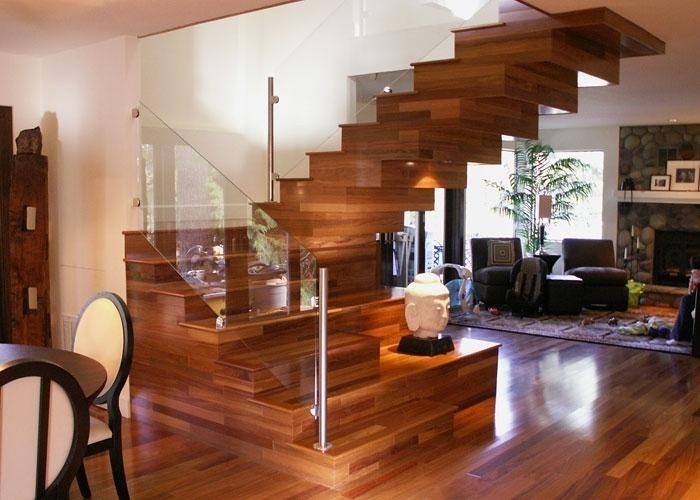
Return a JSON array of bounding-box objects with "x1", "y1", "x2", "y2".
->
[
  {"x1": 470, "y1": 236, "x2": 530, "y2": 310},
  {"x1": 561, "y1": 238, "x2": 629, "y2": 313},
  {"x1": 70, "y1": 291, "x2": 135, "y2": 500},
  {"x1": 504, "y1": 254, "x2": 546, "y2": 316},
  {"x1": 0, "y1": 360, "x2": 92, "y2": 500}
]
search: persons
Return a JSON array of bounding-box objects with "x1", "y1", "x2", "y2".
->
[{"x1": 403, "y1": 272, "x2": 453, "y2": 338}]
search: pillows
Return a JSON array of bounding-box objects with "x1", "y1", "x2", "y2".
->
[{"x1": 486, "y1": 239, "x2": 516, "y2": 266}]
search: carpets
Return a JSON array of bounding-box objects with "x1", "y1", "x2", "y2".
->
[{"x1": 446, "y1": 302, "x2": 693, "y2": 356}]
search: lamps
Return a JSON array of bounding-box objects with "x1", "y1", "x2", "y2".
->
[{"x1": 535, "y1": 189, "x2": 553, "y2": 255}]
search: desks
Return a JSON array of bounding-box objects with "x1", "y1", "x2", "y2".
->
[
  {"x1": 0, "y1": 342, "x2": 108, "y2": 499},
  {"x1": 527, "y1": 253, "x2": 561, "y2": 273}
]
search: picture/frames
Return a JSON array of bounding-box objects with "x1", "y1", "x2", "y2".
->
[
  {"x1": 649, "y1": 173, "x2": 672, "y2": 191},
  {"x1": 665, "y1": 158, "x2": 700, "y2": 191}
]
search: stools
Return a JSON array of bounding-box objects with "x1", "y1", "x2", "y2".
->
[{"x1": 544, "y1": 273, "x2": 584, "y2": 316}]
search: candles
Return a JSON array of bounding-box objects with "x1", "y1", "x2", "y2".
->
[
  {"x1": 636, "y1": 236, "x2": 640, "y2": 249},
  {"x1": 631, "y1": 225, "x2": 635, "y2": 237},
  {"x1": 624, "y1": 247, "x2": 628, "y2": 259}
]
